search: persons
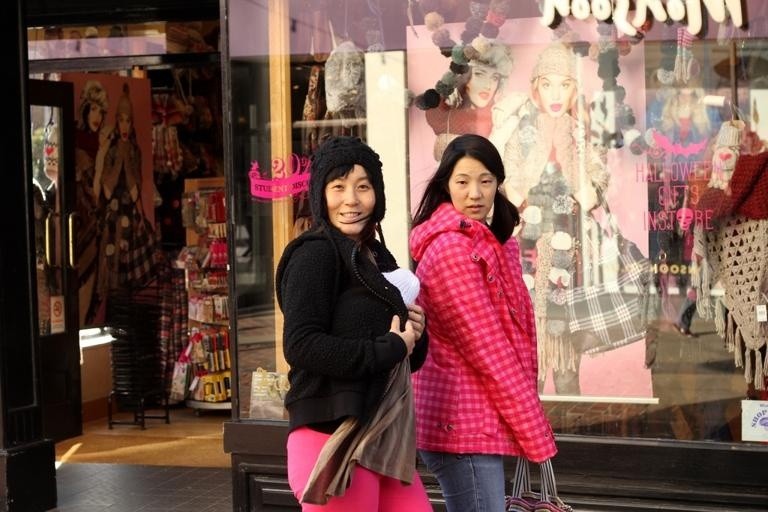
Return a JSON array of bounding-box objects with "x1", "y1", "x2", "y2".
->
[
  {"x1": 98, "y1": 80, "x2": 158, "y2": 291},
  {"x1": 433, "y1": 40, "x2": 512, "y2": 161},
  {"x1": 649, "y1": 191, "x2": 688, "y2": 323},
  {"x1": 503, "y1": 41, "x2": 650, "y2": 398},
  {"x1": 407, "y1": 132, "x2": 560, "y2": 512},
  {"x1": 73, "y1": 77, "x2": 109, "y2": 326},
  {"x1": 670, "y1": 192, "x2": 720, "y2": 341},
  {"x1": 650, "y1": 59, "x2": 725, "y2": 191},
  {"x1": 271, "y1": 132, "x2": 434, "y2": 512}
]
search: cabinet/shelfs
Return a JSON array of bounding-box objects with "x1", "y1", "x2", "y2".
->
[{"x1": 182, "y1": 175, "x2": 235, "y2": 417}]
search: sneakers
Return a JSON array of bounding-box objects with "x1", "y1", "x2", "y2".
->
[{"x1": 673, "y1": 320, "x2": 699, "y2": 339}]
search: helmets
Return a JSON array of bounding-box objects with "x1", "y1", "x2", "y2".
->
[
  {"x1": 476, "y1": 40, "x2": 515, "y2": 75},
  {"x1": 78, "y1": 80, "x2": 109, "y2": 114},
  {"x1": 117, "y1": 84, "x2": 133, "y2": 121},
  {"x1": 529, "y1": 39, "x2": 579, "y2": 84}
]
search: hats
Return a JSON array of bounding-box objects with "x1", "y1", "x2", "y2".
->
[{"x1": 311, "y1": 134, "x2": 385, "y2": 225}]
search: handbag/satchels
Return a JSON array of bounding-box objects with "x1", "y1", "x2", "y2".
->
[{"x1": 502, "y1": 458, "x2": 573, "y2": 512}]
